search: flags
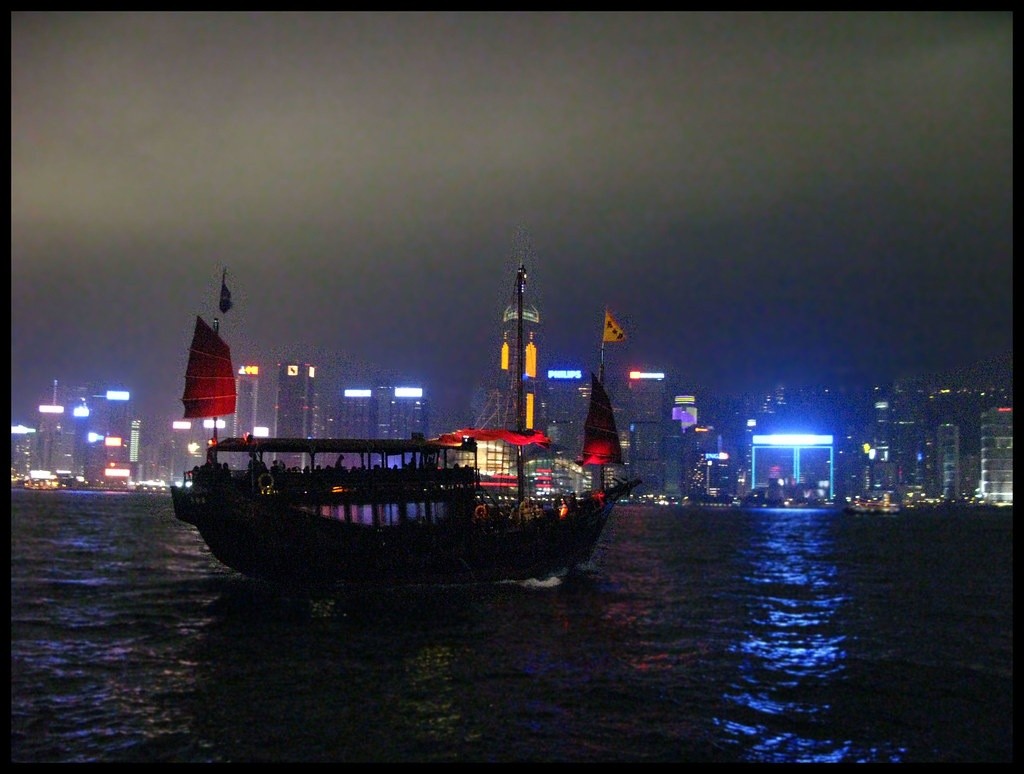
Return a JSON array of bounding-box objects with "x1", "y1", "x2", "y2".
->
[{"x1": 219, "y1": 282, "x2": 232, "y2": 314}]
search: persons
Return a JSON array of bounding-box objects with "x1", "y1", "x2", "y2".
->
[
  {"x1": 223, "y1": 463, "x2": 231, "y2": 476},
  {"x1": 519, "y1": 491, "x2": 605, "y2": 523},
  {"x1": 247, "y1": 453, "x2": 459, "y2": 474}
]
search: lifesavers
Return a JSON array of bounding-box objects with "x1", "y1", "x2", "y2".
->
[
  {"x1": 474, "y1": 505, "x2": 487, "y2": 518},
  {"x1": 257, "y1": 472, "x2": 275, "y2": 490}
]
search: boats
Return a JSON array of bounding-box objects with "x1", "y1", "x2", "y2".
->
[
  {"x1": 845, "y1": 492, "x2": 902, "y2": 515},
  {"x1": 171, "y1": 262, "x2": 645, "y2": 585}
]
